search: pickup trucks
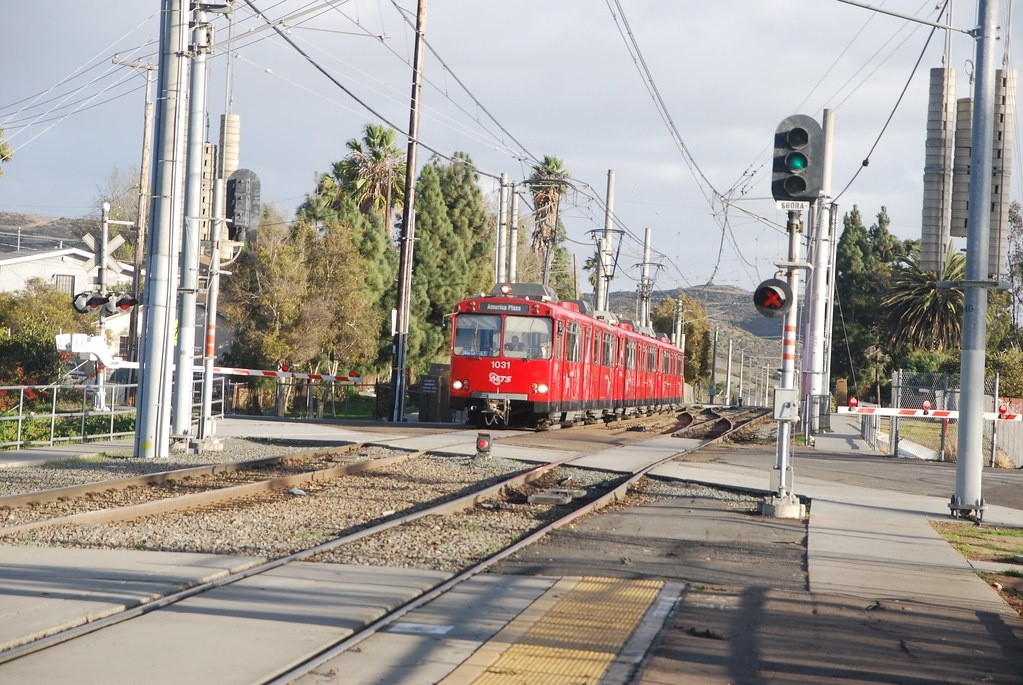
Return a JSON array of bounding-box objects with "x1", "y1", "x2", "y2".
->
[{"x1": 59, "y1": 360, "x2": 86, "y2": 386}]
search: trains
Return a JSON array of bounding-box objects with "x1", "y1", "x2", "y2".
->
[{"x1": 442, "y1": 281, "x2": 686, "y2": 431}]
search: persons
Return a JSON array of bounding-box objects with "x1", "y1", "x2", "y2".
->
[
  {"x1": 739, "y1": 397, "x2": 743, "y2": 407},
  {"x1": 506, "y1": 335, "x2": 524, "y2": 351}
]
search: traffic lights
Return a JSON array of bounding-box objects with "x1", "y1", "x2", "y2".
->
[
  {"x1": 754, "y1": 278, "x2": 793, "y2": 318},
  {"x1": 116, "y1": 292, "x2": 138, "y2": 314},
  {"x1": 86, "y1": 290, "x2": 110, "y2": 314},
  {"x1": 770, "y1": 114, "x2": 823, "y2": 210}
]
